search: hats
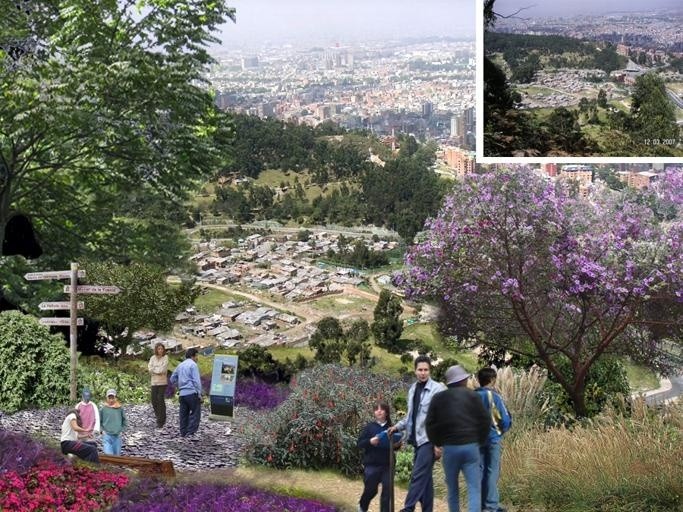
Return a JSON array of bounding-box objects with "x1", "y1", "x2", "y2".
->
[
  {"x1": 106, "y1": 389, "x2": 117, "y2": 398},
  {"x1": 82, "y1": 391, "x2": 92, "y2": 401},
  {"x1": 444, "y1": 365, "x2": 470, "y2": 386}
]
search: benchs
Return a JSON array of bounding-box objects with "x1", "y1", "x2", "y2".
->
[{"x1": 75, "y1": 452, "x2": 177, "y2": 482}]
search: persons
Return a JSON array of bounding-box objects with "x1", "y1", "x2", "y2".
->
[
  {"x1": 61, "y1": 388, "x2": 128, "y2": 465},
  {"x1": 357, "y1": 355, "x2": 511, "y2": 512},
  {"x1": 169, "y1": 348, "x2": 204, "y2": 442},
  {"x1": 148, "y1": 342, "x2": 168, "y2": 429}
]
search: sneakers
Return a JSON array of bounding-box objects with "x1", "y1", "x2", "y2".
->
[
  {"x1": 155, "y1": 422, "x2": 166, "y2": 431},
  {"x1": 185, "y1": 433, "x2": 201, "y2": 441}
]
